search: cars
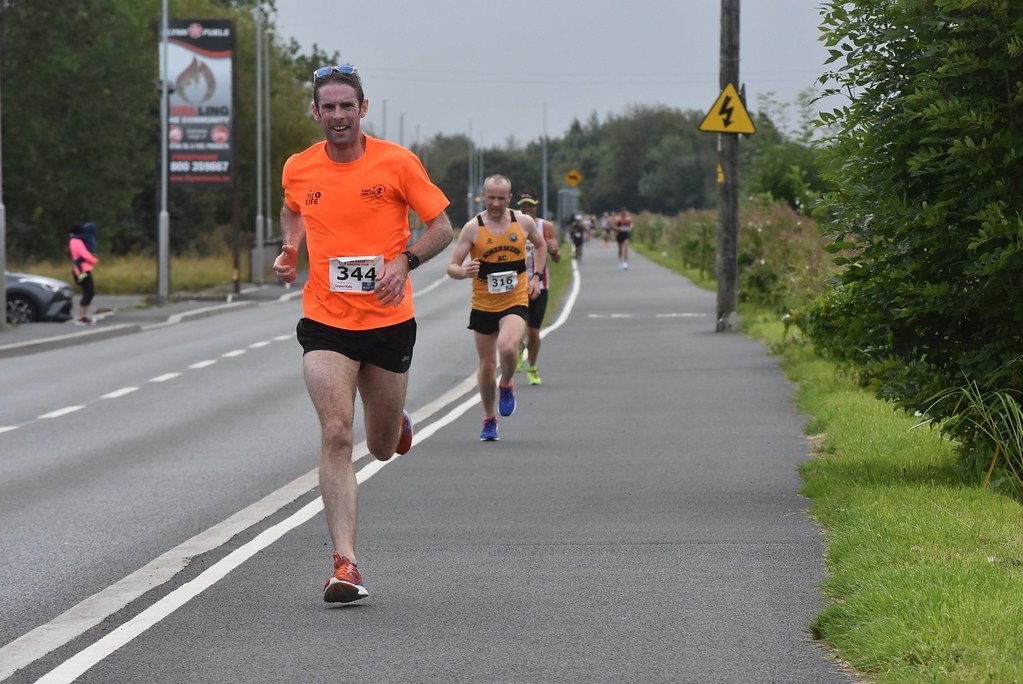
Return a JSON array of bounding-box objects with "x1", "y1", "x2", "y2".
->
[{"x1": 5, "y1": 268, "x2": 74, "y2": 325}]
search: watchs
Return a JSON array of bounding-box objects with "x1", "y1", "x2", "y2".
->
[{"x1": 402, "y1": 251, "x2": 419, "y2": 270}]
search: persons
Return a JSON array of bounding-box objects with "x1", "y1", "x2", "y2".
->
[
  {"x1": 515, "y1": 192, "x2": 560, "y2": 385},
  {"x1": 274, "y1": 64, "x2": 454, "y2": 603},
  {"x1": 609, "y1": 206, "x2": 634, "y2": 269},
  {"x1": 447, "y1": 174, "x2": 547, "y2": 441},
  {"x1": 569, "y1": 214, "x2": 598, "y2": 260},
  {"x1": 69, "y1": 224, "x2": 98, "y2": 324},
  {"x1": 69, "y1": 222, "x2": 96, "y2": 283},
  {"x1": 601, "y1": 212, "x2": 611, "y2": 247}
]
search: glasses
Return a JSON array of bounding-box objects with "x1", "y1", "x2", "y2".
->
[{"x1": 313, "y1": 65, "x2": 362, "y2": 88}]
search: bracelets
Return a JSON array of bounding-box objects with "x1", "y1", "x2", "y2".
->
[
  {"x1": 534, "y1": 272, "x2": 543, "y2": 280},
  {"x1": 551, "y1": 254, "x2": 557, "y2": 256}
]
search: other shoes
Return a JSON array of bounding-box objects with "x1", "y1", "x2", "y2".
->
[
  {"x1": 623, "y1": 262, "x2": 628, "y2": 268},
  {"x1": 77, "y1": 318, "x2": 93, "y2": 325}
]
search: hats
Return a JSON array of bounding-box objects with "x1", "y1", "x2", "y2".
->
[{"x1": 516, "y1": 193, "x2": 537, "y2": 205}]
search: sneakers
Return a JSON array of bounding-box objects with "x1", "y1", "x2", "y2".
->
[
  {"x1": 515, "y1": 342, "x2": 525, "y2": 372},
  {"x1": 323, "y1": 552, "x2": 369, "y2": 604},
  {"x1": 480, "y1": 417, "x2": 499, "y2": 441},
  {"x1": 396, "y1": 409, "x2": 412, "y2": 455},
  {"x1": 527, "y1": 368, "x2": 541, "y2": 385},
  {"x1": 499, "y1": 378, "x2": 516, "y2": 416}
]
